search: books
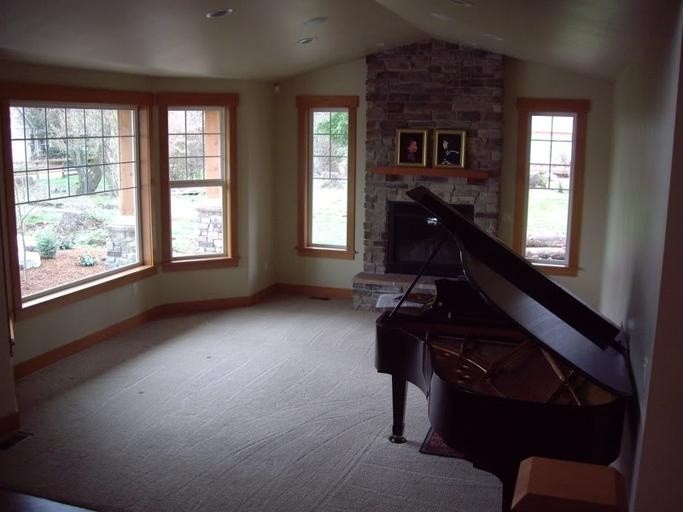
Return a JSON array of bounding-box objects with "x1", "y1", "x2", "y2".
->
[
  {"x1": 374, "y1": 293, "x2": 424, "y2": 309},
  {"x1": 393, "y1": 291, "x2": 435, "y2": 304}
]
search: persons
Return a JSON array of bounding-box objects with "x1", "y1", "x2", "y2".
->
[
  {"x1": 436, "y1": 133, "x2": 461, "y2": 167},
  {"x1": 396, "y1": 132, "x2": 422, "y2": 167}
]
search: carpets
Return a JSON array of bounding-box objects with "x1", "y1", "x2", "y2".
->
[{"x1": 420, "y1": 425, "x2": 462, "y2": 458}]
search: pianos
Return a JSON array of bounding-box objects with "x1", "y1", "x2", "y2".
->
[{"x1": 376, "y1": 186, "x2": 633, "y2": 512}]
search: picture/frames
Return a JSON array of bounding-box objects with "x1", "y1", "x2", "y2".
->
[
  {"x1": 394, "y1": 129, "x2": 427, "y2": 167},
  {"x1": 432, "y1": 129, "x2": 466, "y2": 169}
]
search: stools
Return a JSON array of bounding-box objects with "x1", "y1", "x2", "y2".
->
[{"x1": 502, "y1": 455, "x2": 630, "y2": 512}]
